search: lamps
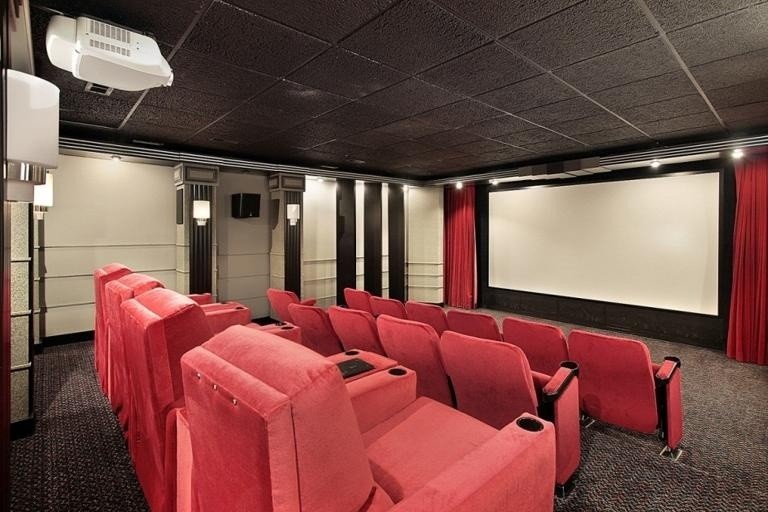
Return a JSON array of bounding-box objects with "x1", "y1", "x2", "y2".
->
[{"x1": 7, "y1": 67, "x2": 60, "y2": 203}]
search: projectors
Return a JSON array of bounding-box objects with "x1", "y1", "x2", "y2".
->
[{"x1": 44, "y1": 12, "x2": 176, "y2": 93}]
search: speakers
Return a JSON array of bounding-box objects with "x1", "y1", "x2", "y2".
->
[{"x1": 230, "y1": 192, "x2": 262, "y2": 219}]
